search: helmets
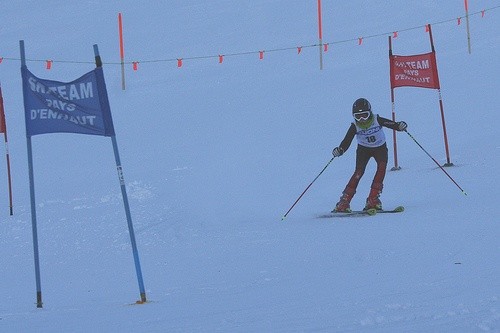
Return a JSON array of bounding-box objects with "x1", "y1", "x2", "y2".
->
[{"x1": 351, "y1": 98, "x2": 374, "y2": 125}]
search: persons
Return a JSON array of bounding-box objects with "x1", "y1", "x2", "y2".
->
[{"x1": 332, "y1": 97, "x2": 408, "y2": 213}]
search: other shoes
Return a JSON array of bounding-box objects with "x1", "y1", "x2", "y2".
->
[
  {"x1": 330, "y1": 185, "x2": 356, "y2": 213},
  {"x1": 362, "y1": 181, "x2": 384, "y2": 213}
]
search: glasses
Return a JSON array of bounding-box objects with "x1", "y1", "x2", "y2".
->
[{"x1": 353, "y1": 111, "x2": 371, "y2": 121}]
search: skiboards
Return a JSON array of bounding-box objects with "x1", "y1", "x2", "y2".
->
[{"x1": 315, "y1": 206, "x2": 404, "y2": 218}]
